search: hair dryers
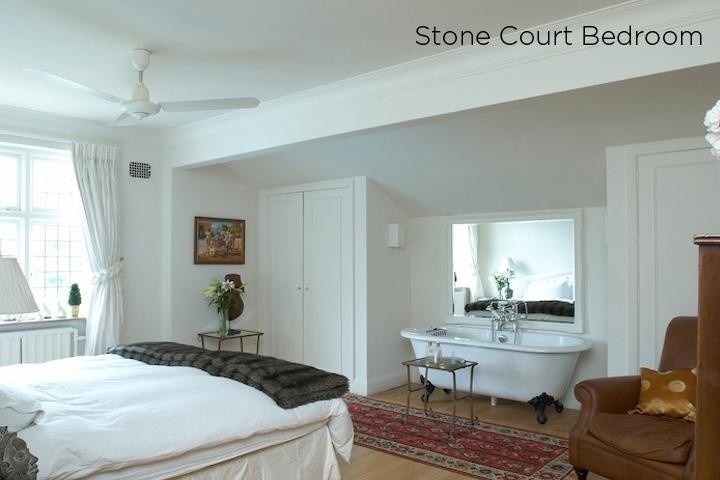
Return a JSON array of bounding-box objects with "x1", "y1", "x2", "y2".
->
[{"x1": 425, "y1": 341, "x2": 434, "y2": 364}]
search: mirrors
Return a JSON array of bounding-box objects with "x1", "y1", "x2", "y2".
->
[{"x1": 445, "y1": 209, "x2": 586, "y2": 335}]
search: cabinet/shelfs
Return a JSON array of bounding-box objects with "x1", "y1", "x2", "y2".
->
[
  {"x1": 260, "y1": 188, "x2": 356, "y2": 378},
  {"x1": 692, "y1": 234, "x2": 720, "y2": 480}
]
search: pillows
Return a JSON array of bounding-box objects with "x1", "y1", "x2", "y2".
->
[
  {"x1": 0, "y1": 367, "x2": 44, "y2": 432},
  {"x1": 628, "y1": 366, "x2": 697, "y2": 422}
]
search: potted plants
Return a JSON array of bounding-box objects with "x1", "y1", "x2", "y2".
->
[{"x1": 67, "y1": 283, "x2": 81, "y2": 316}]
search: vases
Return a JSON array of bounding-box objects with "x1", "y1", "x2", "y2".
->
[
  {"x1": 217, "y1": 307, "x2": 230, "y2": 336},
  {"x1": 496, "y1": 288, "x2": 503, "y2": 301}
]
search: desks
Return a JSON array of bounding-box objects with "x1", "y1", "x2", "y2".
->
[
  {"x1": 401, "y1": 356, "x2": 478, "y2": 439},
  {"x1": 197, "y1": 330, "x2": 263, "y2": 355}
]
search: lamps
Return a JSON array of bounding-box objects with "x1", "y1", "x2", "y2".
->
[{"x1": 1, "y1": 255, "x2": 40, "y2": 321}]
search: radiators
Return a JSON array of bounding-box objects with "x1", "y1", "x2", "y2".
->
[{"x1": 0, "y1": 327, "x2": 79, "y2": 367}]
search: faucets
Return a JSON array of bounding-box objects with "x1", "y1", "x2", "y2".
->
[{"x1": 498, "y1": 316, "x2": 508, "y2": 330}]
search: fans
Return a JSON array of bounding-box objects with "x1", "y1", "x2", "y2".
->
[{"x1": 20, "y1": 46, "x2": 260, "y2": 131}]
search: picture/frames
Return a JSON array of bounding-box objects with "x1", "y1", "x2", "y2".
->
[{"x1": 193, "y1": 216, "x2": 245, "y2": 265}]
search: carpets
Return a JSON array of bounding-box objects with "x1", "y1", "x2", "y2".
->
[{"x1": 343, "y1": 394, "x2": 579, "y2": 480}]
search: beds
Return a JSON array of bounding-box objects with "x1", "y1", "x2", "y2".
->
[
  {"x1": 1, "y1": 342, "x2": 355, "y2": 479},
  {"x1": 465, "y1": 299, "x2": 574, "y2": 324}
]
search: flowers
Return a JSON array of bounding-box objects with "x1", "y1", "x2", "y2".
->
[
  {"x1": 486, "y1": 265, "x2": 516, "y2": 290},
  {"x1": 203, "y1": 277, "x2": 247, "y2": 317}
]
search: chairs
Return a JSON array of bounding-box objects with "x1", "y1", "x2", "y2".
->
[{"x1": 569, "y1": 317, "x2": 698, "y2": 480}]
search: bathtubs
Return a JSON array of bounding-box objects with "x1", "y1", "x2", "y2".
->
[{"x1": 399, "y1": 323, "x2": 593, "y2": 425}]
